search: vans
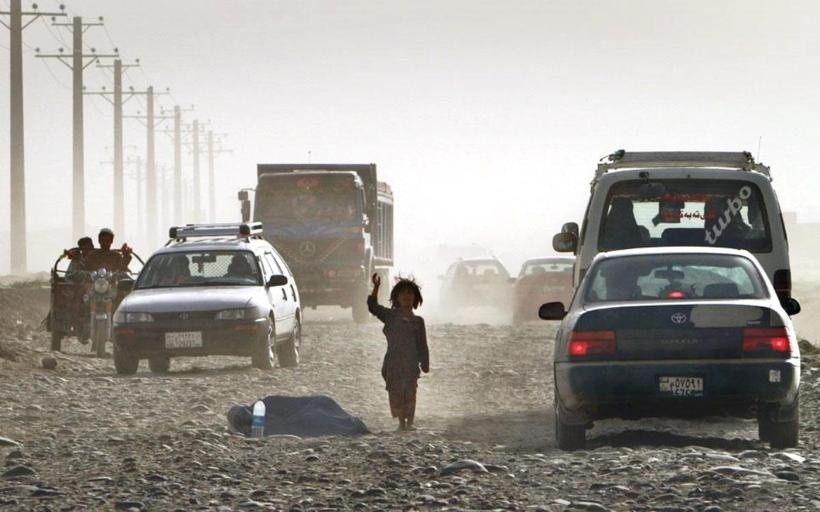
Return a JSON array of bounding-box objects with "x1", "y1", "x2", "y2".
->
[{"x1": 553, "y1": 149, "x2": 792, "y2": 318}]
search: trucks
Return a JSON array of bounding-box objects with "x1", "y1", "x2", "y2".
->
[{"x1": 238, "y1": 163, "x2": 395, "y2": 324}]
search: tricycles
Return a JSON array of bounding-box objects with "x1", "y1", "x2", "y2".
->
[{"x1": 50, "y1": 249, "x2": 145, "y2": 359}]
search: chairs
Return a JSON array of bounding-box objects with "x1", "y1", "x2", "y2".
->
[
  {"x1": 703, "y1": 198, "x2": 752, "y2": 243},
  {"x1": 606, "y1": 275, "x2": 742, "y2": 300},
  {"x1": 606, "y1": 197, "x2": 639, "y2": 246}
]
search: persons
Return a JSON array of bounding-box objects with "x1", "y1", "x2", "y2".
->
[
  {"x1": 68, "y1": 237, "x2": 93, "y2": 281},
  {"x1": 229, "y1": 256, "x2": 254, "y2": 277},
  {"x1": 366, "y1": 274, "x2": 431, "y2": 431},
  {"x1": 91, "y1": 229, "x2": 130, "y2": 280}
]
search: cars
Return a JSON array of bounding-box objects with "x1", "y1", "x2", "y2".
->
[
  {"x1": 436, "y1": 255, "x2": 576, "y2": 326},
  {"x1": 537, "y1": 245, "x2": 801, "y2": 449},
  {"x1": 112, "y1": 220, "x2": 303, "y2": 375}
]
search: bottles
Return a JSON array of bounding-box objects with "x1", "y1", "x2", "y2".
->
[{"x1": 250, "y1": 398, "x2": 266, "y2": 439}]
